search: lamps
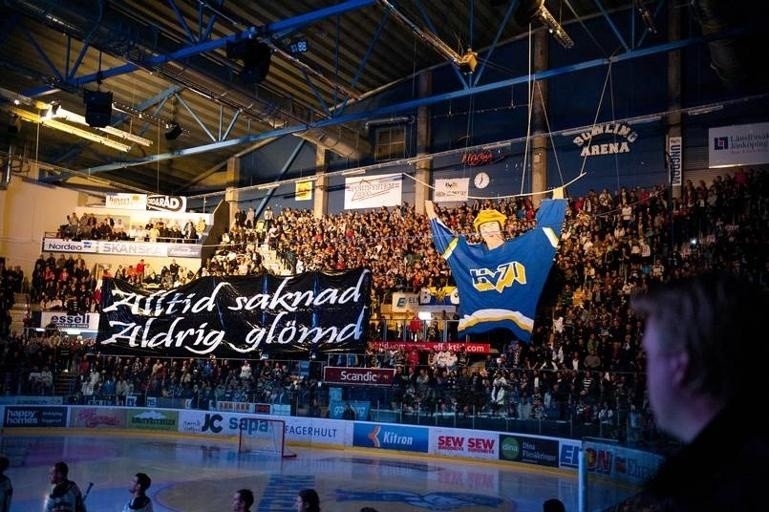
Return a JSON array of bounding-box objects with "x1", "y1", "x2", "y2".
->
[
  {"x1": 45, "y1": 96, "x2": 63, "y2": 115},
  {"x1": 81, "y1": 46, "x2": 116, "y2": 130},
  {"x1": 163, "y1": 120, "x2": 182, "y2": 141},
  {"x1": 457, "y1": 47, "x2": 479, "y2": 73},
  {"x1": 216, "y1": 0, "x2": 275, "y2": 85}
]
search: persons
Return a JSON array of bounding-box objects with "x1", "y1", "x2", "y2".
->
[
  {"x1": 297, "y1": 489, "x2": 321, "y2": 512},
  {"x1": 600, "y1": 269, "x2": 769, "y2": 512},
  {"x1": 0, "y1": 456, "x2": 13, "y2": 512},
  {"x1": 232, "y1": 488, "x2": 254, "y2": 512},
  {"x1": 43, "y1": 461, "x2": 87, "y2": 512},
  {"x1": 122, "y1": 473, "x2": 153, "y2": 512},
  {"x1": 0, "y1": 166, "x2": 769, "y2": 431}
]
